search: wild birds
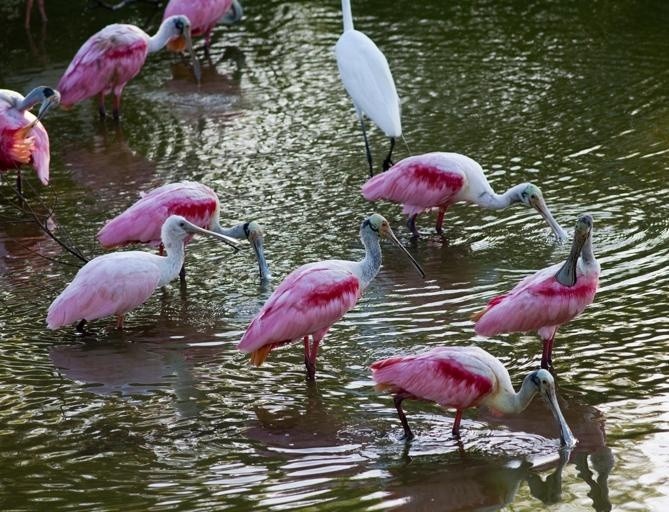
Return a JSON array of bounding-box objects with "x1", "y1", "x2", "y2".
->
[
  {"x1": 161, "y1": 0, "x2": 245, "y2": 60},
  {"x1": 0, "y1": 85, "x2": 62, "y2": 207},
  {"x1": 369, "y1": 342, "x2": 578, "y2": 452},
  {"x1": 475, "y1": 381, "x2": 617, "y2": 512},
  {"x1": 55, "y1": 13, "x2": 202, "y2": 125},
  {"x1": 379, "y1": 441, "x2": 573, "y2": 512},
  {"x1": 43, "y1": 214, "x2": 240, "y2": 333},
  {"x1": 236, "y1": 212, "x2": 426, "y2": 381},
  {"x1": 332, "y1": 0, "x2": 405, "y2": 170},
  {"x1": 472, "y1": 214, "x2": 602, "y2": 369},
  {"x1": 94, "y1": 178, "x2": 269, "y2": 282},
  {"x1": 359, "y1": 150, "x2": 563, "y2": 241}
]
